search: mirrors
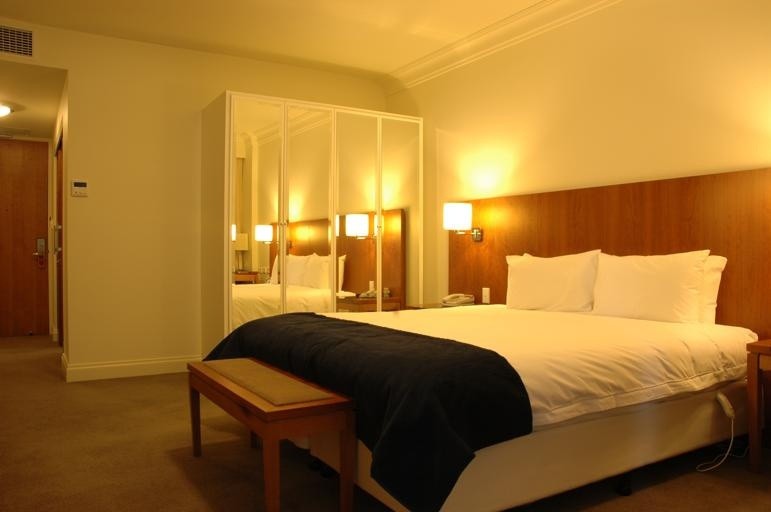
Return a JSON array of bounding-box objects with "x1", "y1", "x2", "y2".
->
[{"x1": 230, "y1": 95, "x2": 424, "y2": 332}]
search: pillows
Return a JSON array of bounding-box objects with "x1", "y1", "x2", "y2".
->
[
  {"x1": 270, "y1": 252, "x2": 347, "y2": 291},
  {"x1": 505, "y1": 250, "x2": 728, "y2": 324}
]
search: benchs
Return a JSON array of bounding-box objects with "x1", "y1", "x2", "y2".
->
[{"x1": 186, "y1": 356, "x2": 357, "y2": 511}]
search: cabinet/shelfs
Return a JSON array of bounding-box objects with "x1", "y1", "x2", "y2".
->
[{"x1": 201, "y1": 88, "x2": 425, "y2": 357}]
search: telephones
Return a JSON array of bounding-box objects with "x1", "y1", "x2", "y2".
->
[
  {"x1": 359, "y1": 289, "x2": 376, "y2": 298},
  {"x1": 441, "y1": 293, "x2": 475, "y2": 306}
]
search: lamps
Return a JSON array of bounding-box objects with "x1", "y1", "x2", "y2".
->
[
  {"x1": 234, "y1": 233, "x2": 249, "y2": 274},
  {"x1": 254, "y1": 224, "x2": 278, "y2": 245},
  {"x1": 443, "y1": 203, "x2": 482, "y2": 242},
  {"x1": 345, "y1": 214, "x2": 384, "y2": 241}
]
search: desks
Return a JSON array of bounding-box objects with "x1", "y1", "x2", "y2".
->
[
  {"x1": 336, "y1": 296, "x2": 402, "y2": 312},
  {"x1": 232, "y1": 272, "x2": 258, "y2": 284},
  {"x1": 746, "y1": 340, "x2": 771, "y2": 474},
  {"x1": 406, "y1": 302, "x2": 487, "y2": 310}
]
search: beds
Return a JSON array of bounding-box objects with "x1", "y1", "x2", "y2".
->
[
  {"x1": 232, "y1": 284, "x2": 356, "y2": 329},
  {"x1": 232, "y1": 303, "x2": 759, "y2": 511}
]
